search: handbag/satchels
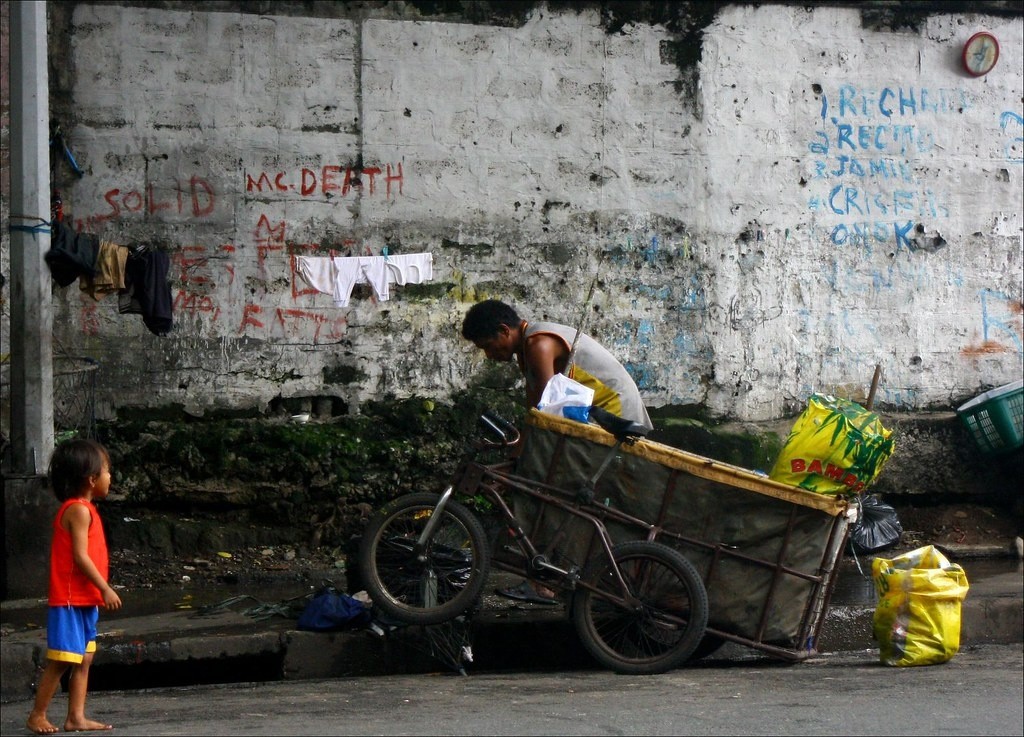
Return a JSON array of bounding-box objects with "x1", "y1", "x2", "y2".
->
[
  {"x1": 296, "y1": 584, "x2": 370, "y2": 632},
  {"x1": 537, "y1": 373, "x2": 596, "y2": 423}
]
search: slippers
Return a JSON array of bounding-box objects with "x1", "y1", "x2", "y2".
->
[{"x1": 494, "y1": 580, "x2": 558, "y2": 605}]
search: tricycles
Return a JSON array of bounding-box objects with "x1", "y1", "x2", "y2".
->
[{"x1": 349, "y1": 403, "x2": 865, "y2": 677}]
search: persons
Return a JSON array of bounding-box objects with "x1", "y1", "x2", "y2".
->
[
  {"x1": 27, "y1": 436, "x2": 122, "y2": 733},
  {"x1": 461, "y1": 301, "x2": 654, "y2": 609}
]
